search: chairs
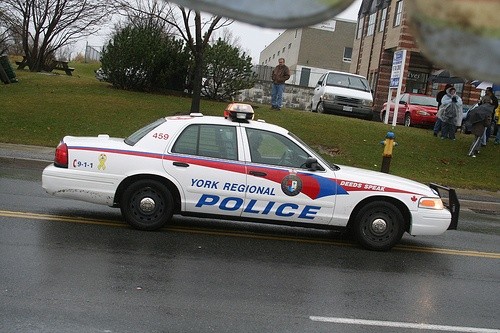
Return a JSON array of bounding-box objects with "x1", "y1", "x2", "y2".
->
[{"x1": 215, "y1": 127, "x2": 235, "y2": 159}]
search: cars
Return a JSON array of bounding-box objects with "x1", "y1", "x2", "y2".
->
[
  {"x1": 309, "y1": 70, "x2": 374, "y2": 118},
  {"x1": 40, "y1": 103, "x2": 453, "y2": 252},
  {"x1": 381, "y1": 92, "x2": 443, "y2": 129},
  {"x1": 95, "y1": 64, "x2": 153, "y2": 90}
]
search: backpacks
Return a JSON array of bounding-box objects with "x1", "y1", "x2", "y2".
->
[
  {"x1": 470, "y1": 105, "x2": 489, "y2": 123},
  {"x1": 436, "y1": 103, "x2": 458, "y2": 121}
]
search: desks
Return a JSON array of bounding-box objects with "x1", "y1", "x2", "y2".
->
[{"x1": 17, "y1": 55, "x2": 72, "y2": 76}]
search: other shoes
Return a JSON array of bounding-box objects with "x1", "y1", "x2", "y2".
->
[
  {"x1": 434, "y1": 130, "x2": 437, "y2": 136},
  {"x1": 450, "y1": 138, "x2": 455, "y2": 140},
  {"x1": 466, "y1": 155, "x2": 476, "y2": 157},
  {"x1": 481, "y1": 143, "x2": 485, "y2": 146},
  {"x1": 495, "y1": 142, "x2": 499, "y2": 145},
  {"x1": 441, "y1": 136, "x2": 447, "y2": 139},
  {"x1": 278, "y1": 107, "x2": 281, "y2": 110},
  {"x1": 476, "y1": 150, "x2": 480, "y2": 154},
  {"x1": 271, "y1": 105, "x2": 277, "y2": 108}
]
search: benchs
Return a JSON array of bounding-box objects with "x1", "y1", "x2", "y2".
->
[
  {"x1": 15, "y1": 61, "x2": 29, "y2": 66},
  {"x1": 55, "y1": 66, "x2": 75, "y2": 71}
]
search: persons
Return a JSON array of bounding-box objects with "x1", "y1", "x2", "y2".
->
[
  {"x1": 480, "y1": 86, "x2": 500, "y2": 145},
  {"x1": 248, "y1": 130, "x2": 264, "y2": 161},
  {"x1": 439, "y1": 88, "x2": 463, "y2": 141},
  {"x1": 268, "y1": 58, "x2": 291, "y2": 112},
  {"x1": 492, "y1": 101, "x2": 500, "y2": 148},
  {"x1": 432, "y1": 82, "x2": 455, "y2": 138},
  {"x1": 465, "y1": 96, "x2": 493, "y2": 159}
]
